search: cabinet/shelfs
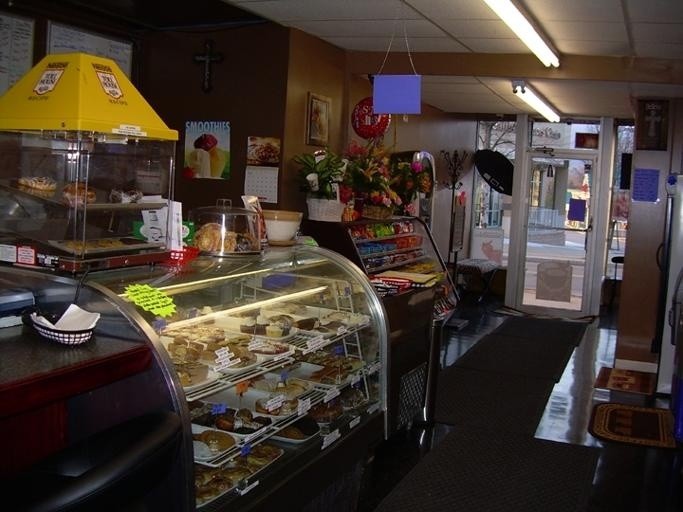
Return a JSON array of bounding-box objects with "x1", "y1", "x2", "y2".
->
[
  {"x1": 308, "y1": 215, "x2": 461, "y2": 451},
  {"x1": 79, "y1": 242, "x2": 387, "y2": 512}
]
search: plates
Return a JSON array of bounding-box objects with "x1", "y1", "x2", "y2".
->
[{"x1": 269, "y1": 241, "x2": 295, "y2": 247}]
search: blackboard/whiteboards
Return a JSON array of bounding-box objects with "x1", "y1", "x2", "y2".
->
[
  {"x1": 46, "y1": 20, "x2": 134, "y2": 84},
  {"x1": 0, "y1": 11, "x2": 35, "y2": 96}
]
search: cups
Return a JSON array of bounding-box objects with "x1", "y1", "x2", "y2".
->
[{"x1": 306, "y1": 173, "x2": 319, "y2": 192}]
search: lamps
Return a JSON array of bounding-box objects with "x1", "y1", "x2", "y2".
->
[
  {"x1": 512, "y1": 79, "x2": 562, "y2": 124},
  {"x1": 483, "y1": 1, "x2": 561, "y2": 69}
]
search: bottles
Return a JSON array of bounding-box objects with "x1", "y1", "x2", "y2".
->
[{"x1": 342, "y1": 204, "x2": 358, "y2": 222}]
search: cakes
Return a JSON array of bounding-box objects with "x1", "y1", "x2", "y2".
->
[
  {"x1": 312, "y1": 387, "x2": 365, "y2": 423},
  {"x1": 249, "y1": 374, "x2": 311, "y2": 414},
  {"x1": 235, "y1": 232, "x2": 259, "y2": 252},
  {"x1": 240, "y1": 315, "x2": 293, "y2": 337}
]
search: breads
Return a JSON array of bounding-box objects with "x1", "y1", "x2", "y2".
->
[
  {"x1": 190, "y1": 400, "x2": 320, "y2": 497},
  {"x1": 110, "y1": 188, "x2": 142, "y2": 202},
  {"x1": 194, "y1": 223, "x2": 236, "y2": 252},
  {"x1": 17, "y1": 174, "x2": 58, "y2": 192},
  {"x1": 63, "y1": 181, "x2": 96, "y2": 203},
  {"x1": 66, "y1": 238, "x2": 148, "y2": 250},
  {"x1": 167, "y1": 325, "x2": 289, "y2": 386}
]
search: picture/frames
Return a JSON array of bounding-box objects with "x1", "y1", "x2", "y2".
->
[{"x1": 304, "y1": 93, "x2": 332, "y2": 148}]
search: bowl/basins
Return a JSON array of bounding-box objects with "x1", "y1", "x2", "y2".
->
[{"x1": 262, "y1": 210, "x2": 302, "y2": 240}]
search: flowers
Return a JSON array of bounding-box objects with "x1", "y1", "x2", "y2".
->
[{"x1": 293, "y1": 153, "x2": 431, "y2": 208}]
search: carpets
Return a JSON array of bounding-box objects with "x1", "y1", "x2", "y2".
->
[
  {"x1": 304, "y1": 422, "x2": 602, "y2": 511},
  {"x1": 431, "y1": 314, "x2": 585, "y2": 435}
]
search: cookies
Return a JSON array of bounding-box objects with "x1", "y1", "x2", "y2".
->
[{"x1": 310, "y1": 354, "x2": 364, "y2": 383}]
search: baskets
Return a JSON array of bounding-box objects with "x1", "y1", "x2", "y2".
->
[
  {"x1": 25, "y1": 308, "x2": 93, "y2": 347},
  {"x1": 305, "y1": 182, "x2": 347, "y2": 223},
  {"x1": 360, "y1": 203, "x2": 395, "y2": 220}
]
